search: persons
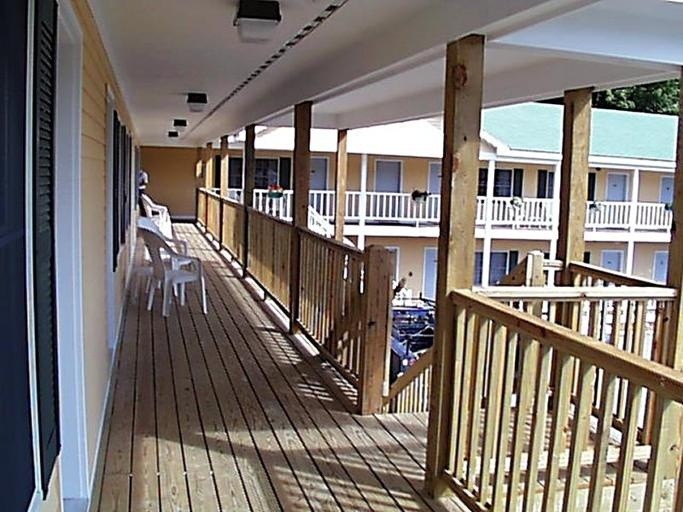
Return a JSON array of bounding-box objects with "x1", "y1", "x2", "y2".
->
[{"x1": 138, "y1": 168, "x2": 149, "y2": 216}]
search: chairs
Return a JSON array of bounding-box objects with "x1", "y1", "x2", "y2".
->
[
  {"x1": 136, "y1": 219, "x2": 208, "y2": 317},
  {"x1": 140, "y1": 193, "x2": 173, "y2": 235},
  {"x1": 136, "y1": 215, "x2": 188, "y2": 297}
]
